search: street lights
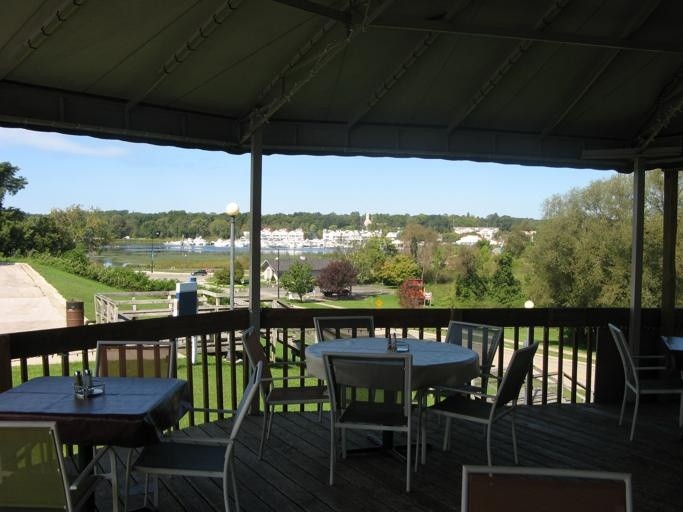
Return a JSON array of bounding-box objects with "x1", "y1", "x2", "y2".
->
[
  {"x1": 523, "y1": 301, "x2": 534, "y2": 404},
  {"x1": 226, "y1": 203, "x2": 239, "y2": 312},
  {"x1": 150, "y1": 232, "x2": 160, "y2": 272}
]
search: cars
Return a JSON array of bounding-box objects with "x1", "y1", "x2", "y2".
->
[{"x1": 192, "y1": 270, "x2": 207, "y2": 276}]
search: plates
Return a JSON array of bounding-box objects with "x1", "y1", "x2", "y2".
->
[{"x1": 78, "y1": 387, "x2": 103, "y2": 396}]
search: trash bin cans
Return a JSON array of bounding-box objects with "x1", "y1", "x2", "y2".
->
[{"x1": 66, "y1": 301, "x2": 84, "y2": 327}]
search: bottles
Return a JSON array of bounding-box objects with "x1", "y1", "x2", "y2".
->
[{"x1": 73, "y1": 369, "x2": 92, "y2": 393}]
